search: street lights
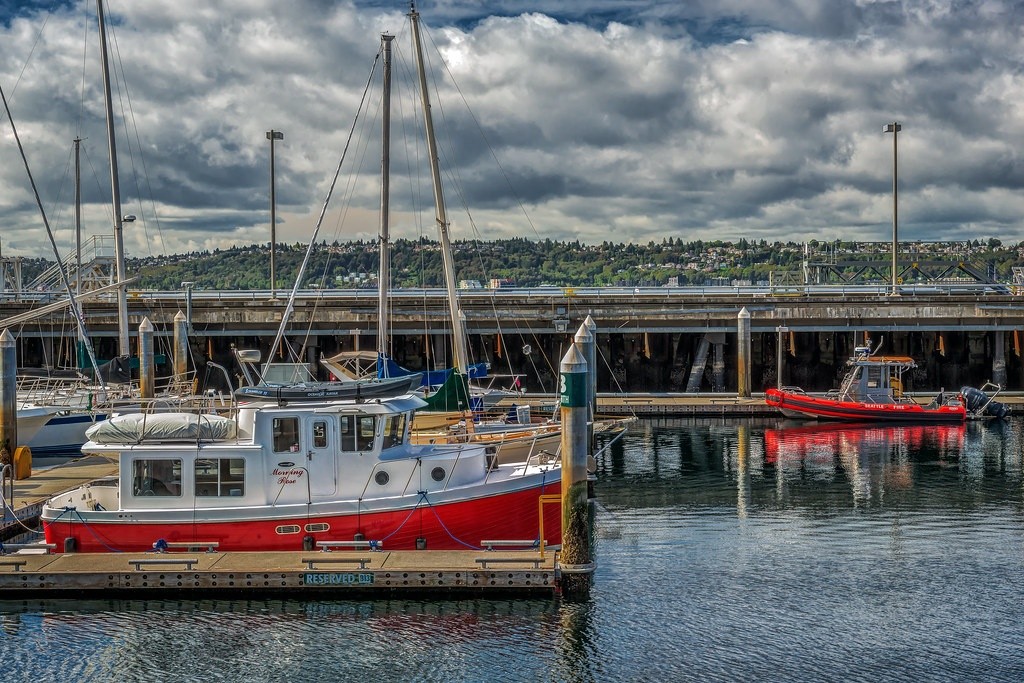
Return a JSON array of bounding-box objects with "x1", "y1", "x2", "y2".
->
[
  {"x1": 883, "y1": 121, "x2": 902, "y2": 296},
  {"x1": 265, "y1": 127, "x2": 283, "y2": 298}
]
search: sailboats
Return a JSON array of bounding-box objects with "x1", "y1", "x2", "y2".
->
[{"x1": 1, "y1": 0, "x2": 642, "y2": 463}]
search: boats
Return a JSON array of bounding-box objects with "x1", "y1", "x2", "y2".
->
[
  {"x1": 37, "y1": 374, "x2": 566, "y2": 553},
  {"x1": 765, "y1": 336, "x2": 1013, "y2": 423}
]
